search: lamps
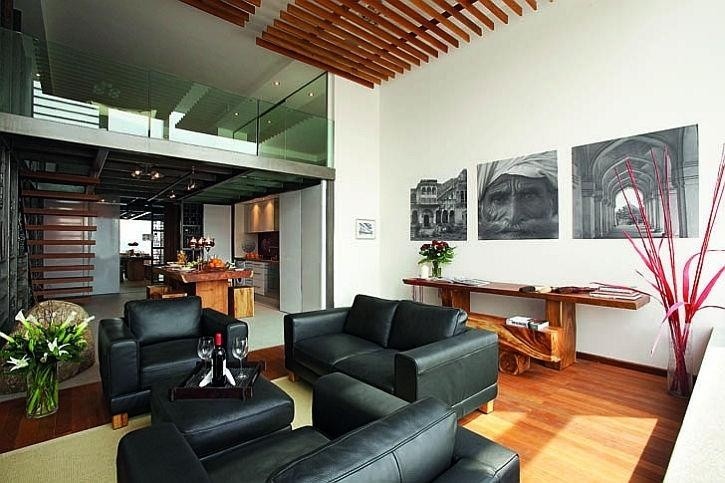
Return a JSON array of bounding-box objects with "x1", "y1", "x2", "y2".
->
[{"x1": 130, "y1": 163, "x2": 196, "y2": 198}]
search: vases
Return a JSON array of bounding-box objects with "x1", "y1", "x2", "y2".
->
[
  {"x1": 26, "y1": 357, "x2": 60, "y2": 420},
  {"x1": 432, "y1": 262, "x2": 442, "y2": 280}
]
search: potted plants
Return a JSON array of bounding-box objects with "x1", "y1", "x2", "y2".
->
[{"x1": 616, "y1": 138, "x2": 724, "y2": 398}]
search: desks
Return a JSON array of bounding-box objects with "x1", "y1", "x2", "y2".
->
[
  {"x1": 402, "y1": 275, "x2": 650, "y2": 374},
  {"x1": 120, "y1": 252, "x2": 153, "y2": 282}
]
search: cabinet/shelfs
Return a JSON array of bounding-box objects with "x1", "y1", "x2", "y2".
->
[
  {"x1": 263, "y1": 198, "x2": 280, "y2": 232},
  {"x1": 244, "y1": 201, "x2": 263, "y2": 232}
]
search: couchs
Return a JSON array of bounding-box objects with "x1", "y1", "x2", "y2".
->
[{"x1": 284, "y1": 293, "x2": 499, "y2": 421}]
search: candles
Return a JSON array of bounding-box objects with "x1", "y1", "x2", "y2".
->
[
  {"x1": 198, "y1": 236, "x2": 205, "y2": 244},
  {"x1": 206, "y1": 237, "x2": 213, "y2": 243},
  {"x1": 191, "y1": 236, "x2": 197, "y2": 243}
]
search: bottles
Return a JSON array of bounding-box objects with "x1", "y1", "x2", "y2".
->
[{"x1": 212, "y1": 333, "x2": 226, "y2": 385}]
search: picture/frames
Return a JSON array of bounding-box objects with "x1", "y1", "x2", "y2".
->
[{"x1": 353, "y1": 217, "x2": 377, "y2": 239}]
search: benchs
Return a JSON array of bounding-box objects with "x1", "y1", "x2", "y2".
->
[{"x1": 464, "y1": 310, "x2": 565, "y2": 373}]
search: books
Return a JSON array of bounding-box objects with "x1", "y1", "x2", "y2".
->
[
  {"x1": 505, "y1": 316, "x2": 551, "y2": 333},
  {"x1": 433, "y1": 274, "x2": 490, "y2": 287},
  {"x1": 588, "y1": 285, "x2": 643, "y2": 301}
]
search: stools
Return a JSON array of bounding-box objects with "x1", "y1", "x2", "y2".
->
[
  {"x1": 154, "y1": 291, "x2": 186, "y2": 301},
  {"x1": 148, "y1": 371, "x2": 294, "y2": 465},
  {"x1": 146, "y1": 284, "x2": 166, "y2": 299},
  {"x1": 228, "y1": 284, "x2": 256, "y2": 316}
]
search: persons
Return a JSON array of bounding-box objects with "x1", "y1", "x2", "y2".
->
[{"x1": 476, "y1": 150, "x2": 559, "y2": 240}]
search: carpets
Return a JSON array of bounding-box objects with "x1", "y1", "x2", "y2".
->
[{"x1": 0, "y1": 373, "x2": 316, "y2": 481}]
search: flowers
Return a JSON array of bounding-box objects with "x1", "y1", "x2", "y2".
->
[
  {"x1": 0, "y1": 305, "x2": 96, "y2": 413},
  {"x1": 417, "y1": 236, "x2": 457, "y2": 274}
]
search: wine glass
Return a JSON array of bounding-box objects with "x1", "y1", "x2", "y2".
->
[
  {"x1": 231, "y1": 336, "x2": 250, "y2": 379},
  {"x1": 198, "y1": 336, "x2": 214, "y2": 377}
]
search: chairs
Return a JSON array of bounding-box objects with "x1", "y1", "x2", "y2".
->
[
  {"x1": 115, "y1": 370, "x2": 522, "y2": 482},
  {"x1": 98, "y1": 294, "x2": 250, "y2": 429}
]
